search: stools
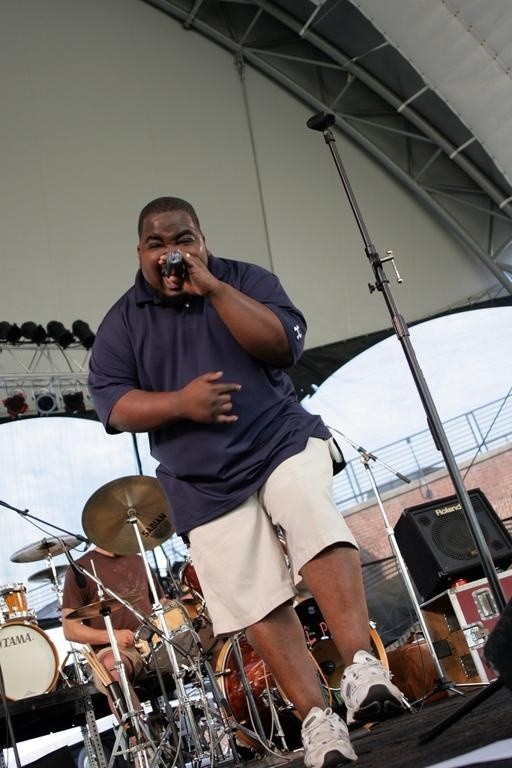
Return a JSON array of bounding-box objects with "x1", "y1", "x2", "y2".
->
[{"x1": 134, "y1": 673, "x2": 176, "y2": 719}]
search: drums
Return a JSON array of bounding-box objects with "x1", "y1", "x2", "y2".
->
[
  {"x1": 135, "y1": 599, "x2": 217, "y2": 680},
  {"x1": 180, "y1": 553, "x2": 206, "y2": 600},
  {"x1": 292, "y1": 592, "x2": 389, "y2": 714},
  {"x1": 211, "y1": 626, "x2": 333, "y2": 754},
  {"x1": 0, "y1": 618, "x2": 60, "y2": 700}
]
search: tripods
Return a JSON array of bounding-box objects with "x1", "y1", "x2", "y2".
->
[
  {"x1": 308, "y1": 109, "x2": 511, "y2": 746},
  {"x1": 0, "y1": 502, "x2": 303, "y2": 768},
  {"x1": 328, "y1": 425, "x2": 492, "y2": 709}
]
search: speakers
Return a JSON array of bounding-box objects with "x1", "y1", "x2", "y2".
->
[{"x1": 395, "y1": 489, "x2": 512, "y2": 601}]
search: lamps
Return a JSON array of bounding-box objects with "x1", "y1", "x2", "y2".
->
[
  {"x1": 2, "y1": 391, "x2": 85, "y2": 421},
  {"x1": 0, "y1": 319, "x2": 95, "y2": 351}
]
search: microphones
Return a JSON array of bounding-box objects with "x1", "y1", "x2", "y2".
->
[{"x1": 165, "y1": 250, "x2": 184, "y2": 279}]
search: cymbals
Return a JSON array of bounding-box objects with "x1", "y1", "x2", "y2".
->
[
  {"x1": 80, "y1": 475, "x2": 176, "y2": 556},
  {"x1": 10, "y1": 534, "x2": 84, "y2": 563},
  {"x1": 29, "y1": 564, "x2": 71, "y2": 586}
]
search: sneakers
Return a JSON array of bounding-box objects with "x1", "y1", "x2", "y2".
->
[
  {"x1": 299, "y1": 705, "x2": 359, "y2": 768},
  {"x1": 339, "y1": 649, "x2": 411, "y2": 727}
]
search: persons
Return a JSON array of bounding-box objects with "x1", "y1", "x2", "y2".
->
[
  {"x1": 62, "y1": 546, "x2": 257, "y2": 768},
  {"x1": 90, "y1": 196, "x2": 411, "y2": 768}
]
